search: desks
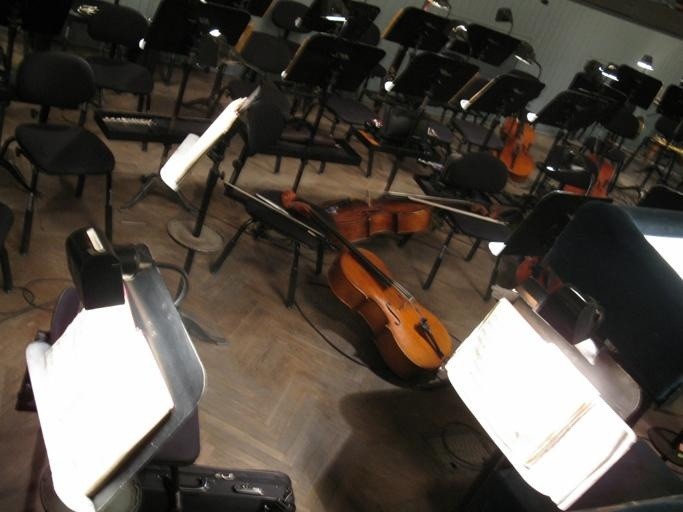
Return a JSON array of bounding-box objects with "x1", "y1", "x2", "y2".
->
[{"x1": 541, "y1": 203, "x2": 683, "y2": 436}]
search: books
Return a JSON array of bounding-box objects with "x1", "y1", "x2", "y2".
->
[
  {"x1": 176, "y1": 97, "x2": 245, "y2": 184},
  {"x1": 443, "y1": 298, "x2": 639, "y2": 512},
  {"x1": 45, "y1": 279, "x2": 175, "y2": 497}
]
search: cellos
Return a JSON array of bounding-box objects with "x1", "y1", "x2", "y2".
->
[
  {"x1": 563, "y1": 134, "x2": 619, "y2": 199},
  {"x1": 317, "y1": 195, "x2": 502, "y2": 247},
  {"x1": 489, "y1": 102, "x2": 536, "y2": 181},
  {"x1": 280, "y1": 189, "x2": 452, "y2": 380}
]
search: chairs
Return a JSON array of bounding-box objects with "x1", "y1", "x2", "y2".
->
[
  {"x1": 36, "y1": 285, "x2": 206, "y2": 475},
  {"x1": 1, "y1": 0, "x2": 681, "y2": 310}
]
